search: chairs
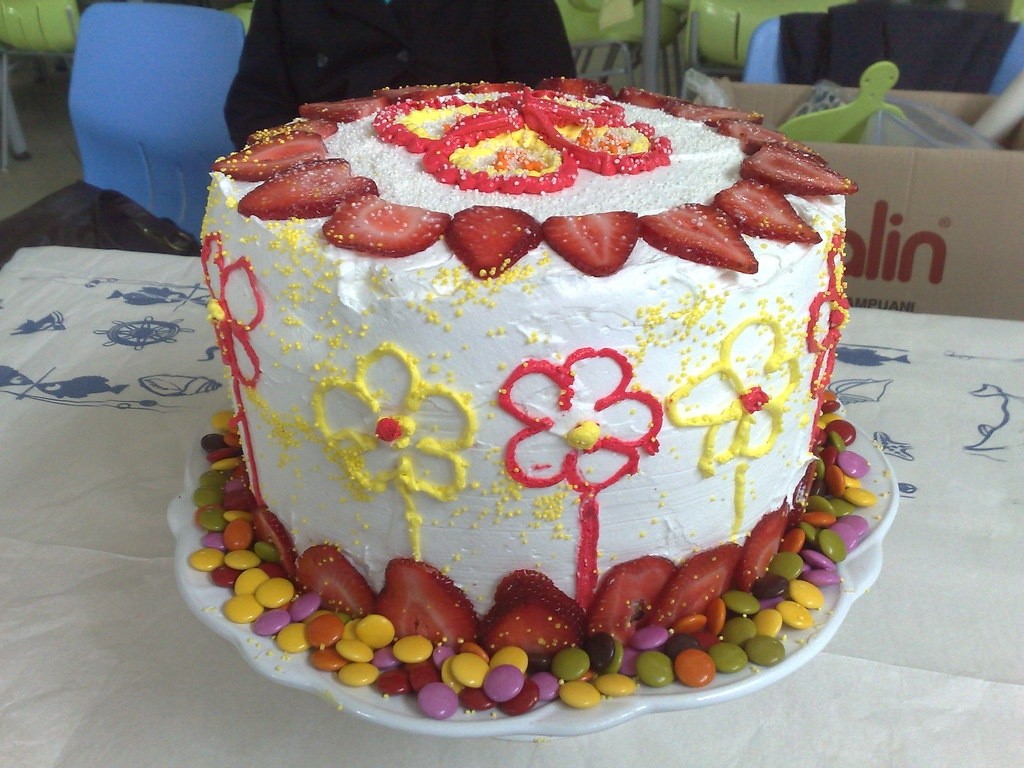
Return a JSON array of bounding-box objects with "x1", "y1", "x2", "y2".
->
[{"x1": 0, "y1": 0, "x2": 1024, "y2": 243}]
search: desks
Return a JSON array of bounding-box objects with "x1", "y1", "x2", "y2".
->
[{"x1": 1, "y1": 241, "x2": 1024, "y2": 767}]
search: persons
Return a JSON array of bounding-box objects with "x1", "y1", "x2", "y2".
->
[{"x1": 223, "y1": 0, "x2": 577, "y2": 154}]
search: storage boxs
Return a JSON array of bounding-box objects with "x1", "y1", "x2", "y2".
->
[{"x1": 695, "y1": 76, "x2": 1023, "y2": 320}]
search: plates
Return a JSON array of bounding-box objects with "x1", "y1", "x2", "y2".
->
[{"x1": 167, "y1": 410, "x2": 899, "y2": 738}]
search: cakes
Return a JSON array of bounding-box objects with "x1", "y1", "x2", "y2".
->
[{"x1": 185, "y1": 77, "x2": 876, "y2": 721}]
